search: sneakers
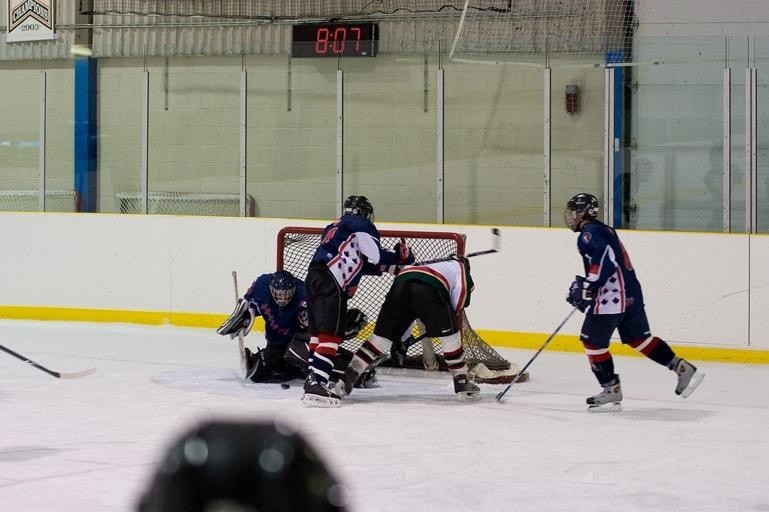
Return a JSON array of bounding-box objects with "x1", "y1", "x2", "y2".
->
[
  {"x1": 304, "y1": 373, "x2": 343, "y2": 401},
  {"x1": 340, "y1": 367, "x2": 359, "y2": 398},
  {"x1": 452, "y1": 373, "x2": 481, "y2": 394}
]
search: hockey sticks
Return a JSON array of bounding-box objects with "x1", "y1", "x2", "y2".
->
[
  {"x1": 233, "y1": 270, "x2": 258, "y2": 388},
  {"x1": 411, "y1": 229, "x2": 502, "y2": 266},
  {"x1": 0, "y1": 343, "x2": 99, "y2": 380},
  {"x1": 472, "y1": 304, "x2": 578, "y2": 401}
]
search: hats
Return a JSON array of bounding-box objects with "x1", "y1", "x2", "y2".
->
[
  {"x1": 671, "y1": 357, "x2": 696, "y2": 396},
  {"x1": 581, "y1": 376, "x2": 623, "y2": 404}
]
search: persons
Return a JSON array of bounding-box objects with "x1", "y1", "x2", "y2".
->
[
  {"x1": 304, "y1": 196, "x2": 413, "y2": 397},
  {"x1": 341, "y1": 256, "x2": 480, "y2": 394},
  {"x1": 247, "y1": 271, "x2": 376, "y2": 387},
  {"x1": 565, "y1": 193, "x2": 696, "y2": 405}
]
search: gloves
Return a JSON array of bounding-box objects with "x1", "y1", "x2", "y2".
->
[
  {"x1": 393, "y1": 242, "x2": 415, "y2": 266},
  {"x1": 566, "y1": 275, "x2": 598, "y2": 314}
]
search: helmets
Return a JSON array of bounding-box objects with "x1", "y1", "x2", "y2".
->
[
  {"x1": 268, "y1": 269, "x2": 297, "y2": 307},
  {"x1": 563, "y1": 193, "x2": 600, "y2": 232},
  {"x1": 344, "y1": 195, "x2": 375, "y2": 224}
]
